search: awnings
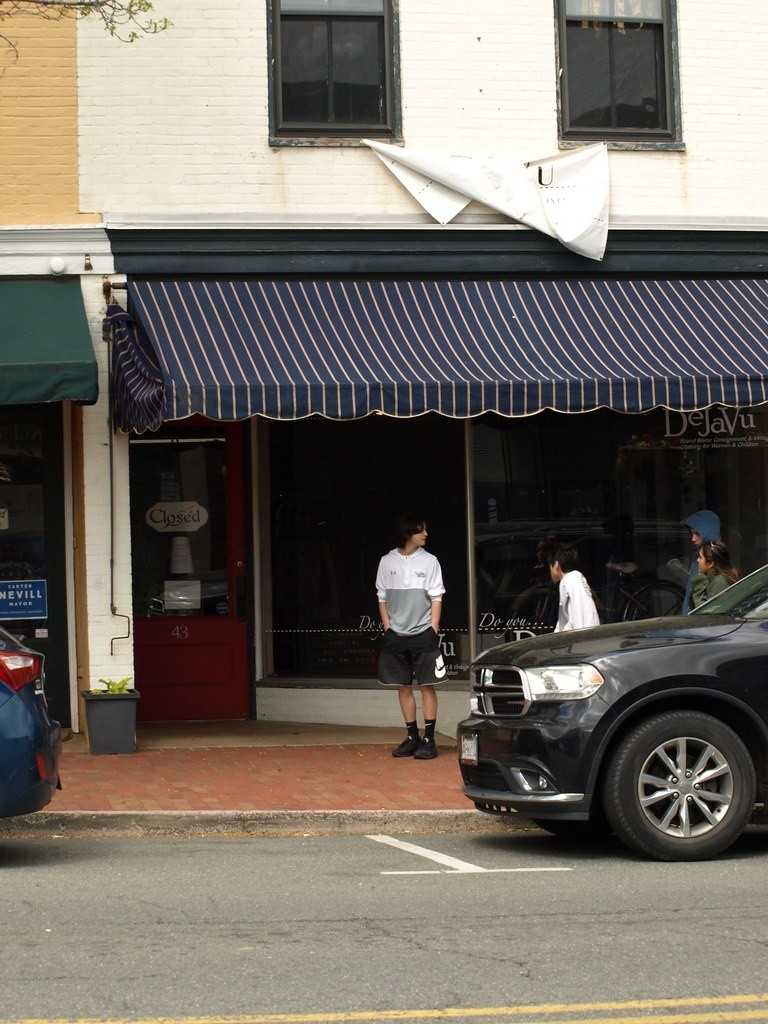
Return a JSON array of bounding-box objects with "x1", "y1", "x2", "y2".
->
[
  {"x1": 101, "y1": 230, "x2": 768, "y2": 435},
  {"x1": 1, "y1": 276, "x2": 100, "y2": 406}
]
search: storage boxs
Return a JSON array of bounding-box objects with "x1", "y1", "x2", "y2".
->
[{"x1": 455, "y1": 632, "x2": 505, "y2": 680}]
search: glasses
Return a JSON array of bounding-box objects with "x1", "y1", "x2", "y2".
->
[{"x1": 708, "y1": 540, "x2": 716, "y2": 550}]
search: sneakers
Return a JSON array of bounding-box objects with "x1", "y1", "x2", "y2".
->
[
  {"x1": 392, "y1": 734, "x2": 421, "y2": 757},
  {"x1": 414, "y1": 736, "x2": 437, "y2": 759}
]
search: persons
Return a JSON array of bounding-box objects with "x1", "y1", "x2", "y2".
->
[
  {"x1": 375, "y1": 515, "x2": 447, "y2": 759},
  {"x1": 682, "y1": 511, "x2": 721, "y2": 616},
  {"x1": 548, "y1": 543, "x2": 601, "y2": 634},
  {"x1": 689, "y1": 540, "x2": 742, "y2": 613}
]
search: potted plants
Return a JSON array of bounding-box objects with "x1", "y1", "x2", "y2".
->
[{"x1": 81, "y1": 678, "x2": 141, "y2": 755}]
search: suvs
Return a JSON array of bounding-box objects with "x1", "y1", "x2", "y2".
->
[{"x1": 457, "y1": 565, "x2": 768, "y2": 863}]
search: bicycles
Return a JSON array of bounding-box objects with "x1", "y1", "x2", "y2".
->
[{"x1": 505, "y1": 564, "x2": 686, "y2": 643}]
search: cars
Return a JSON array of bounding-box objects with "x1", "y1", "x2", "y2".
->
[
  {"x1": 0, "y1": 626, "x2": 63, "y2": 817},
  {"x1": 147, "y1": 570, "x2": 227, "y2": 617}
]
